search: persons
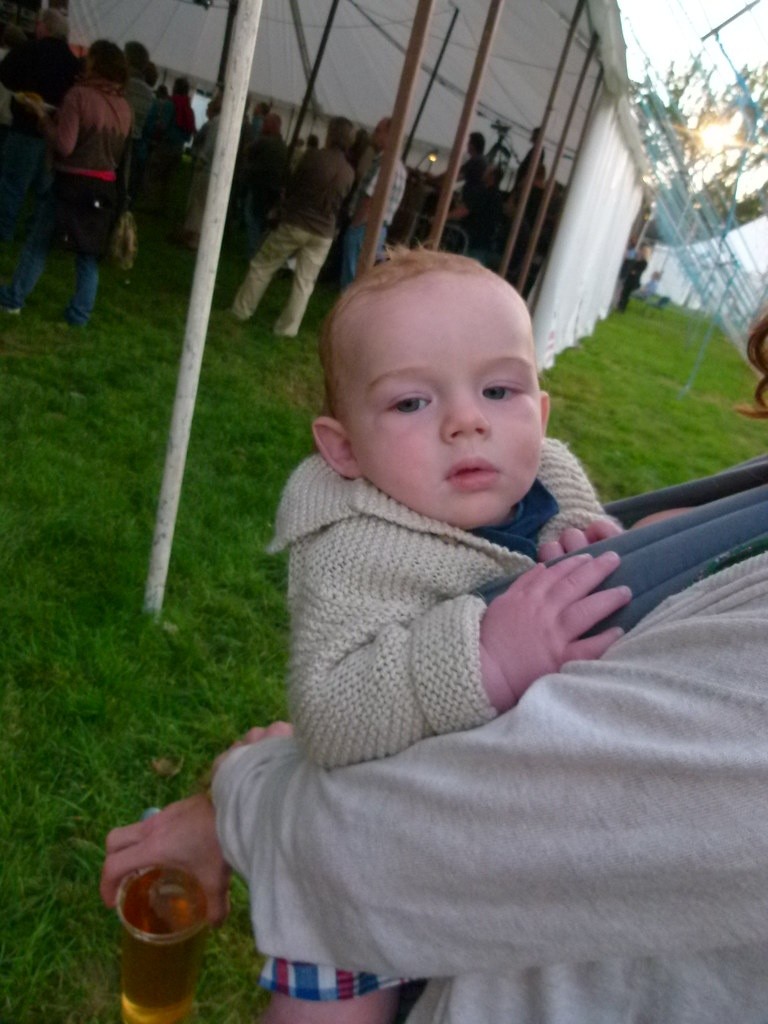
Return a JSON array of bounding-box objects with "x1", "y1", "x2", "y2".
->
[
  {"x1": 0, "y1": 0, "x2": 653, "y2": 339},
  {"x1": 100, "y1": 248, "x2": 768, "y2": 1024}
]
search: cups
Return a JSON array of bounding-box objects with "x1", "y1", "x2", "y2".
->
[{"x1": 114, "y1": 864, "x2": 209, "y2": 1024}]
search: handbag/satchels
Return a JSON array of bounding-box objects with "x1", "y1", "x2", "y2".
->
[{"x1": 109, "y1": 209, "x2": 137, "y2": 270}]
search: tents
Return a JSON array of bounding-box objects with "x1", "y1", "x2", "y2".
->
[{"x1": 35, "y1": 1, "x2": 768, "y2": 376}]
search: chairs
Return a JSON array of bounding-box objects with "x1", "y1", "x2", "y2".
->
[{"x1": 642, "y1": 296, "x2": 670, "y2": 321}]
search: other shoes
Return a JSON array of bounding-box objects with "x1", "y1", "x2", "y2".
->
[
  {"x1": 0, "y1": 304, "x2": 21, "y2": 314},
  {"x1": 176, "y1": 237, "x2": 198, "y2": 251}
]
